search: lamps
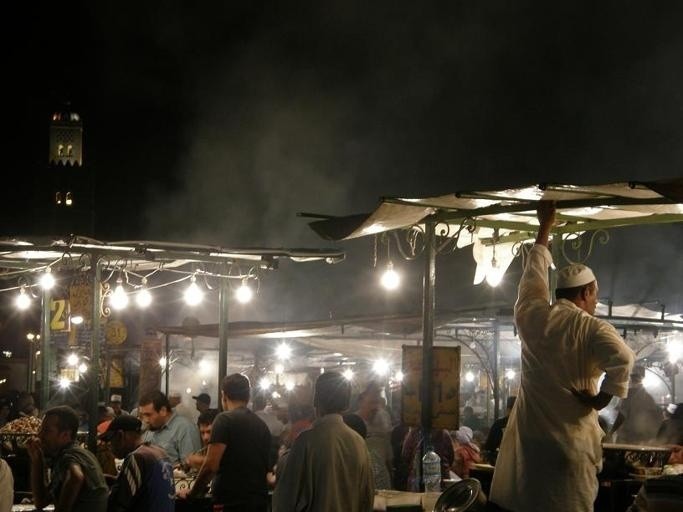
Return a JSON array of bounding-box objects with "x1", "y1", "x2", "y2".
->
[
  {"x1": 378, "y1": 231, "x2": 402, "y2": 295},
  {"x1": 11, "y1": 251, "x2": 261, "y2": 311},
  {"x1": 485, "y1": 237, "x2": 505, "y2": 290}
]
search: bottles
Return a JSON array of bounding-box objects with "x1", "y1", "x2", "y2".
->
[{"x1": 420, "y1": 444, "x2": 442, "y2": 492}]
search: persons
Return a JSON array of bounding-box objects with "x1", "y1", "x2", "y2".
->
[
  {"x1": 602, "y1": 365, "x2": 664, "y2": 443},
  {"x1": 488, "y1": 200, "x2": 633, "y2": 512},
  {"x1": 343, "y1": 380, "x2": 518, "y2": 492},
  {"x1": 0, "y1": 371, "x2": 375, "y2": 510}
]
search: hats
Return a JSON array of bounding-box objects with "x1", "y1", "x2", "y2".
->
[
  {"x1": 192, "y1": 394, "x2": 209, "y2": 404},
  {"x1": 96, "y1": 416, "x2": 141, "y2": 443},
  {"x1": 110, "y1": 395, "x2": 121, "y2": 403},
  {"x1": 556, "y1": 265, "x2": 595, "y2": 289},
  {"x1": 456, "y1": 426, "x2": 474, "y2": 444}
]
